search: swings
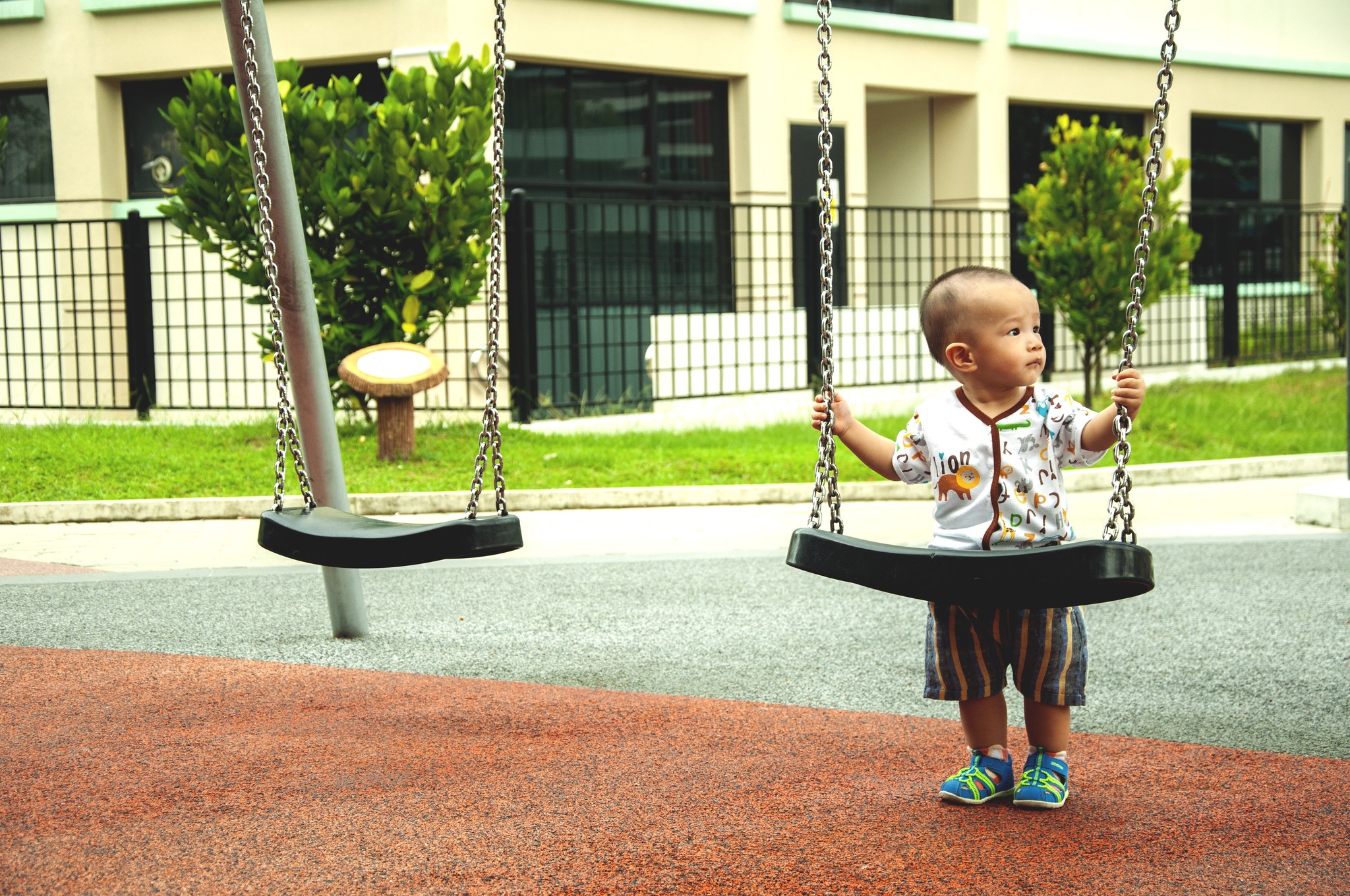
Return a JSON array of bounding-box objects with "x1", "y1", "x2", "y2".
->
[
  {"x1": 784, "y1": 1, "x2": 1183, "y2": 612},
  {"x1": 235, "y1": 0, "x2": 523, "y2": 567}
]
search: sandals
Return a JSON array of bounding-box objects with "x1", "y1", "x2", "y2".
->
[
  {"x1": 938, "y1": 749, "x2": 1016, "y2": 805},
  {"x1": 1013, "y1": 746, "x2": 1069, "y2": 808}
]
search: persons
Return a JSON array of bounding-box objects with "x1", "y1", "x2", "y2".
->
[{"x1": 813, "y1": 266, "x2": 1146, "y2": 808}]
